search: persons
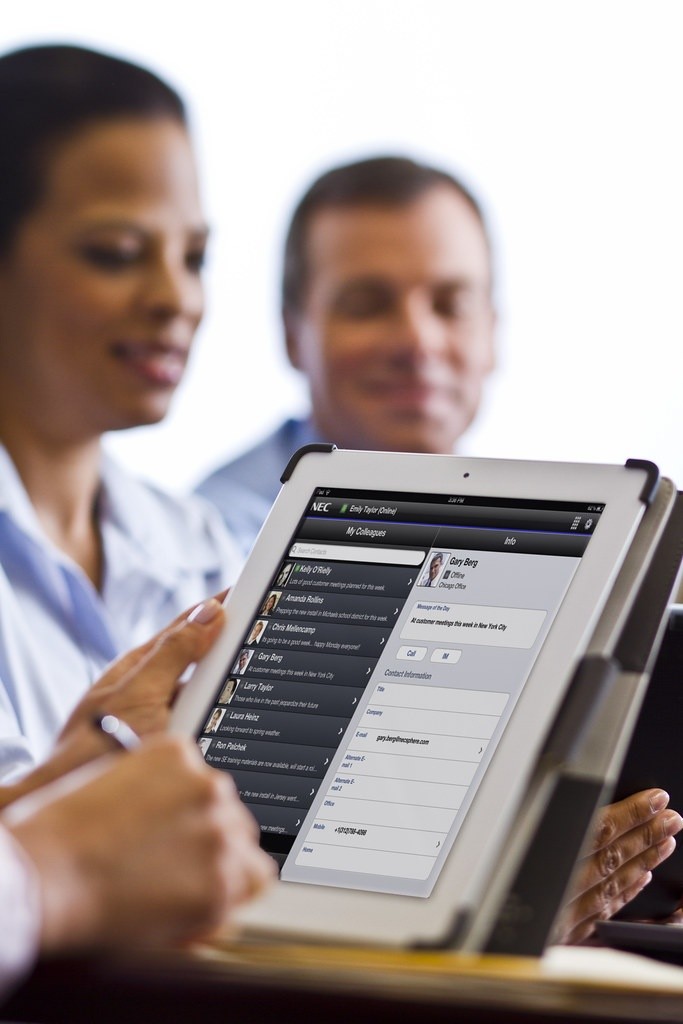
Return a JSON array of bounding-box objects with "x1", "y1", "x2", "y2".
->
[{"x1": 0, "y1": 35, "x2": 682, "y2": 1024}]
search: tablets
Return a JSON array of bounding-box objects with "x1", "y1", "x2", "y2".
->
[{"x1": 163, "y1": 446, "x2": 655, "y2": 955}]
